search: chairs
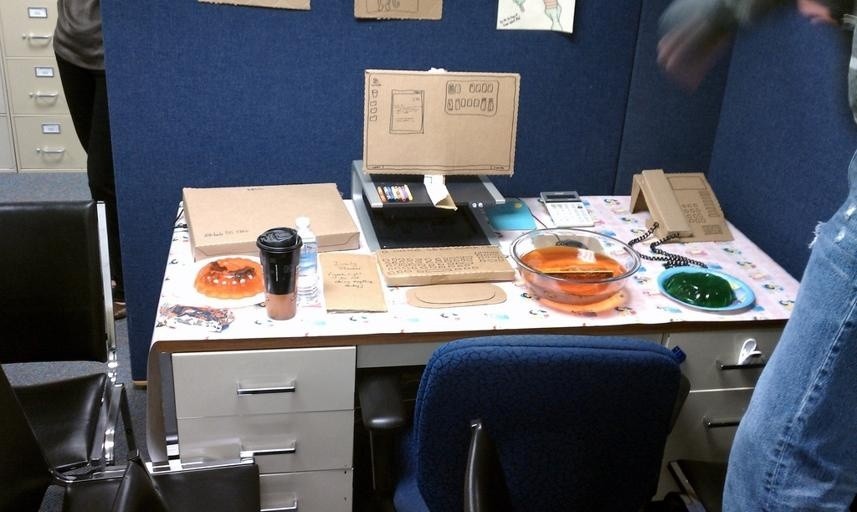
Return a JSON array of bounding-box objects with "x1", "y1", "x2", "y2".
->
[
  {"x1": 354, "y1": 335, "x2": 682, "y2": 511},
  {"x1": 0, "y1": 200, "x2": 138, "y2": 472},
  {"x1": 0, "y1": 365, "x2": 265, "y2": 512},
  {"x1": 111, "y1": 448, "x2": 178, "y2": 511}
]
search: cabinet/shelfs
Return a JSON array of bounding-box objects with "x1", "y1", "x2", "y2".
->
[{"x1": 1, "y1": 1, "x2": 90, "y2": 175}]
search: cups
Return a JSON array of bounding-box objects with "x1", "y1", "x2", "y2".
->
[{"x1": 254, "y1": 227, "x2": 301, "y2": 321}]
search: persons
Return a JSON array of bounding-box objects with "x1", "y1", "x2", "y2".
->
[
  {"x1": 657, "y1": 0, "x2": 854, "y2": 511},
  {"x1": 49, "y1": 0, "x2": 126, "y2": 322}
]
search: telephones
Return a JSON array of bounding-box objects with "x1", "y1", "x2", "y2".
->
[{"x1": 629, "y1": 168, "x2": 734, "y2": 244}]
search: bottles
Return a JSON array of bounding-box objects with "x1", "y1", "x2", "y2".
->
[{"x1": 292, "y1": 216, "x2": 320, "y2": 304}]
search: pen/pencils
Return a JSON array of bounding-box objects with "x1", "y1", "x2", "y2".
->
[{"x1": 377, "y1": 183, "x2": 413, "y2": 203}]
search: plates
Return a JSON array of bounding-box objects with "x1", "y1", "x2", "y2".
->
[
  {"x1": 176, "y1": 253, "x2": 268, "y2": 308},
  {"x1": 656, "y1": 266, "x2": 759, "y2": 313}
]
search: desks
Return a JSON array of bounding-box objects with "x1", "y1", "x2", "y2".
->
[{"x1": 145, "y1": 196, "x2": 800, "y2": 511}]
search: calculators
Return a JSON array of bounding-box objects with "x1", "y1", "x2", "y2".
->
[{"x1": 540, "y1": 190, "x2": 596, "y2": 228}]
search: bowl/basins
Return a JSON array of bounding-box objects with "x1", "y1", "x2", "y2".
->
[{"x1": 509, "y1": 226, "x2": 642, "y2": 307}]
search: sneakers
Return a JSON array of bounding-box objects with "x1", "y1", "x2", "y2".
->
[{"x1": 112, "y1": 300, "x2": 127, "y2": 319}]
